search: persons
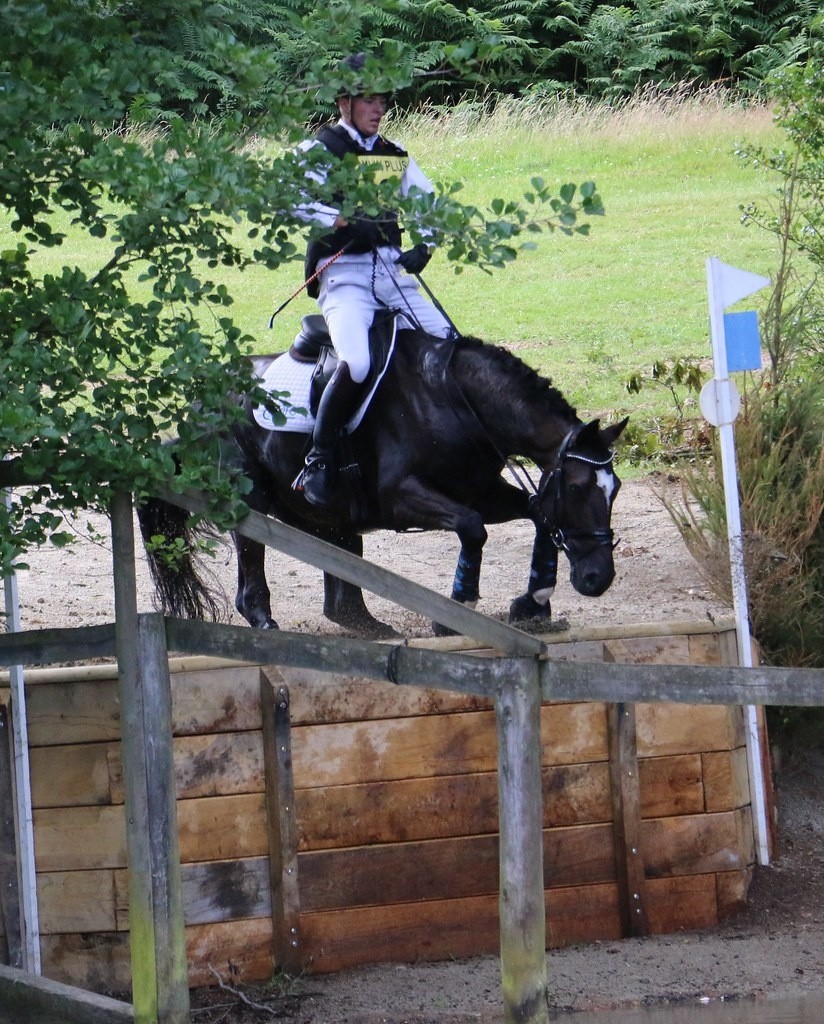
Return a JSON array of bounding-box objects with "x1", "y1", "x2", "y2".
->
[{"x1": 269, "y1": 52, "x2": 461, "y2": 512}]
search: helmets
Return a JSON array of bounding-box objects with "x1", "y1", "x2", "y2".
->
[{"x1": 330, "y1": 53, "x2": 394, "y2": 98}]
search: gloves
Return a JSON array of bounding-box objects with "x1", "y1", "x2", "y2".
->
[
  {"x1": 343, "y1": 210, "x2": 379, "y2": 242},
  {"x1": 393, "y1": 244, "x2": 431, "y2": 274}
]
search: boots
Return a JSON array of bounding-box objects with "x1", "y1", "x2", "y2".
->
[{"x1": 302, "y1": 360, "x2": 373, "y2": 508}]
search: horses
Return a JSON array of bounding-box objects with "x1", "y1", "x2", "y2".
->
[{"x1": 133, "y1": 328, "x2": 632, "y2": 641}]
